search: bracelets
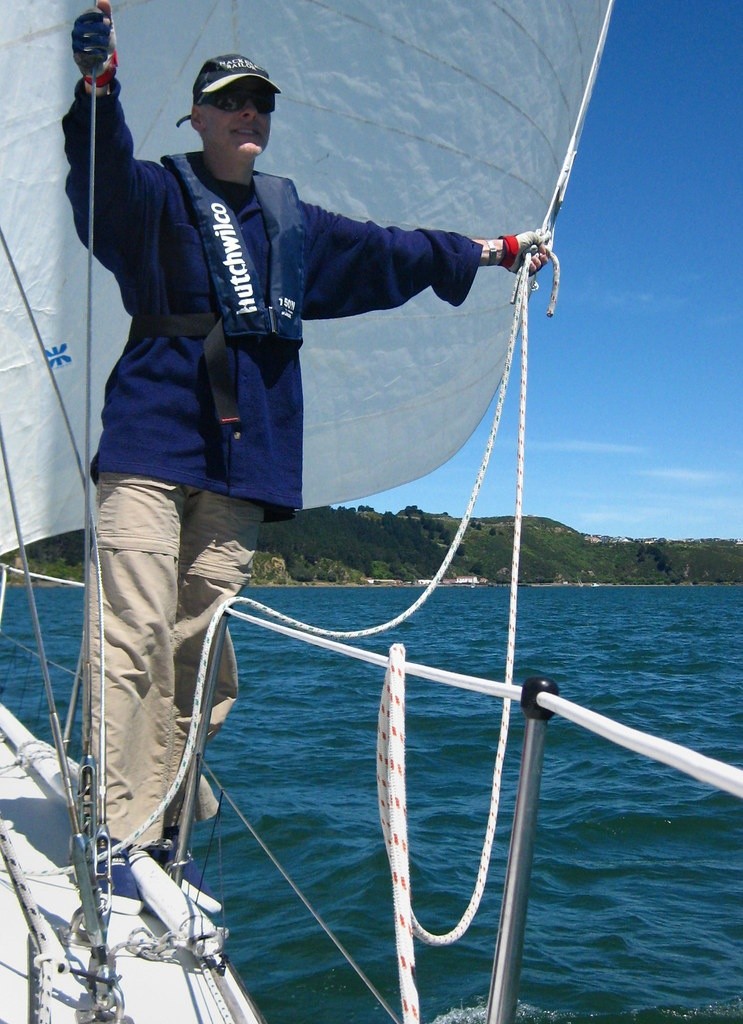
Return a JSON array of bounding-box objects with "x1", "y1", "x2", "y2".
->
[{"x1": 485, "y1": 240, "x2": 496, "y2": 265}]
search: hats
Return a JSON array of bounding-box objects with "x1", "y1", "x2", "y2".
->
[{"x1": 193, "y1": 54, "x2": 281, "y2": 104}]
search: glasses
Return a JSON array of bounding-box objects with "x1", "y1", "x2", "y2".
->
[{"x1": 197, "y1": 87, "x2": 275, "y2": 113}]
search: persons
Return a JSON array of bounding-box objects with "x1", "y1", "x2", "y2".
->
[{"x1": 62, "y1": 0, "x2": 556, "y2": 917}]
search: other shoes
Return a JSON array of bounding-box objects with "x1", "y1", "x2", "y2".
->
[
  {"x1": 147, "y1": 827, "x2": 224, "y2": 913},
  {"x1": 95, "y1": 839, "x2": 146, "y2": 915}
]
